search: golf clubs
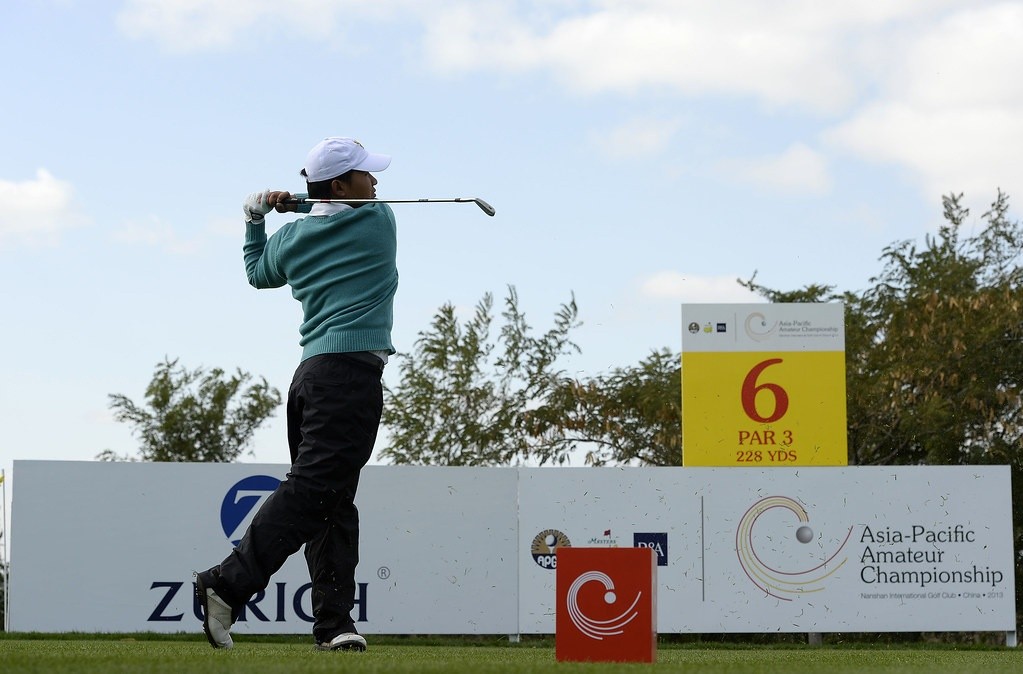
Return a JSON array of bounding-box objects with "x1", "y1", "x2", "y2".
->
[{"x1": 259, "y1": 195, "x2": 497, "y2": 218}]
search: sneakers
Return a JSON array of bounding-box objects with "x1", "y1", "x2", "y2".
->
[
  {"x1": 314, "y1": 623, "x2": 368, "y2": 652},
  {"x1": 196, "y1": 566, "x2": 239, "y2": 649}
]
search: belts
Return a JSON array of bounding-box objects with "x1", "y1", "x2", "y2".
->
[{"x1": 342, "y1": 352, "x2": 384, "y2": 369}]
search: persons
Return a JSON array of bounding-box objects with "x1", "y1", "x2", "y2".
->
[{"x1": 196, "y1": 137, "x2": 398, "y2": 653}]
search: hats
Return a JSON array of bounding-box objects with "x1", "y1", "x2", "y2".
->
[{"x1": 303, "y1": 137, "x2": 390, "y2": 182}]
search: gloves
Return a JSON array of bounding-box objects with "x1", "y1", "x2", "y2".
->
[{"x1": 242, "y1": 190, "x2": 274, "y2": 224}]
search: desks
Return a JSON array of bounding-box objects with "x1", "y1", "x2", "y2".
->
[{"x1": 555, "y1": 547, "x2": 658, "y2": 665}]
